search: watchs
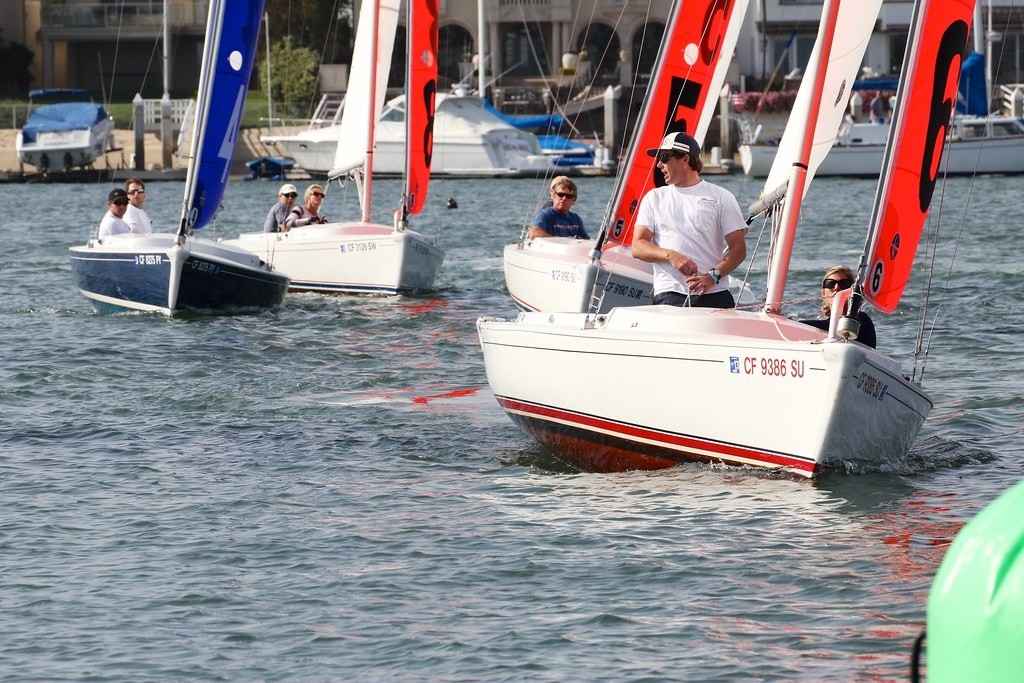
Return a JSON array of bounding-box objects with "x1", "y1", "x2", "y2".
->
[{"x1": 712, "y1": 268, "x2": 721, "y2": 280}]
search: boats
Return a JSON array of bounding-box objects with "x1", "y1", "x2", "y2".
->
[
  {"x1": 261, "y1": 82, "x2": 596, "y2": 177},
  {"x1": 15, "y1": 85, "x2": 116, "y2": 173}
]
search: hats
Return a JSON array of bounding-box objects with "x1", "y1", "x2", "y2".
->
[
  {"x1": 279, "y1": 184, "x2": 299, "y2": 196},
  {"x1": 646, "y1": 132, "x2": 700, "y2": 156},
  {"x1": 109, "y1": 188, "x2": 131, "y2": 203}
]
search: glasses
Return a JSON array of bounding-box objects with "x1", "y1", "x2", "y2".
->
[
  {"x1": 822, "y1": 279, "x2": 851, "y2": 289},
  {"x1": 111, "y1": 200, "x2": 128, "y2": 206},
  {"x1": 555, "y1": 191, "x2": 574, "y2": 199},
  {"x1": 127, "y1": 189, "x2": 145, "y2": 195},
  {"x1": 282, "y1": 192, "x2": 297, "y2": 198},
  {"x1": 658, "y1": 152, "x2": 679, "y2": 163},
  {"x1": 310, "y1": 191, "x2": 324, "y2": 199}
]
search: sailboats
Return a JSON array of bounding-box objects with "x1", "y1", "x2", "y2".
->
[
  {"x1": 501, "y1": 1, "x2": 757, "y2": 313},
  {"x1": 67, "y1": 1, "x2": 292, "y2": 316},
  {"x1": 460, "y1": 1, "x2": 593, "y2": 155},
  {"x1": 217, "y1": 0, "x2": 451, "y2": 296},
  {"x1": 738, "y1": 0, "x2": 1024, "y2": 176},
  {"x1": 474, "y1": 1, "x2": 979, "y2": 483},
  {"x1": 245, "y1": 11, "x2": 294, "y2": 177}
]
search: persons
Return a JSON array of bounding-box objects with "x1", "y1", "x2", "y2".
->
[
  {"x1": 263, "y1": 184, "x2": 298, "y2": 232},
  {"x1": 631, "y1": 132, "x2": 749, "y2": 309},
  {"x1": 869, "y1": 90, "x2": 884, "y2": 124},
  {"x1": 888, "y1": 91, "x2": 896, "y2": 116},
  {"x1": 543, "y1": 176, "x2": 570, "y2": 210},
  {"x1": 529, "y1": 179, "x2": 591, "y2": 241},
  {"x1": 98, "y1": 188, "x2": 132, "y2": 239},
  {"x1": 122, "y1": 177, "x2": 154, "y2": 233},
  {"x1": 284, "y1": 184, "x2": 328, "y2": 228},
  {"x1": 798, "y1": 266, "x2": 877, "y2": 350},
  {"x1": 850, "y1": 90, "x2": 863, "y2": 124}
]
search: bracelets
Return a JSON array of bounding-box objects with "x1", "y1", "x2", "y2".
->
[{"x1": 709, "y1": 271, "x2": 719, "y2": 284}]
